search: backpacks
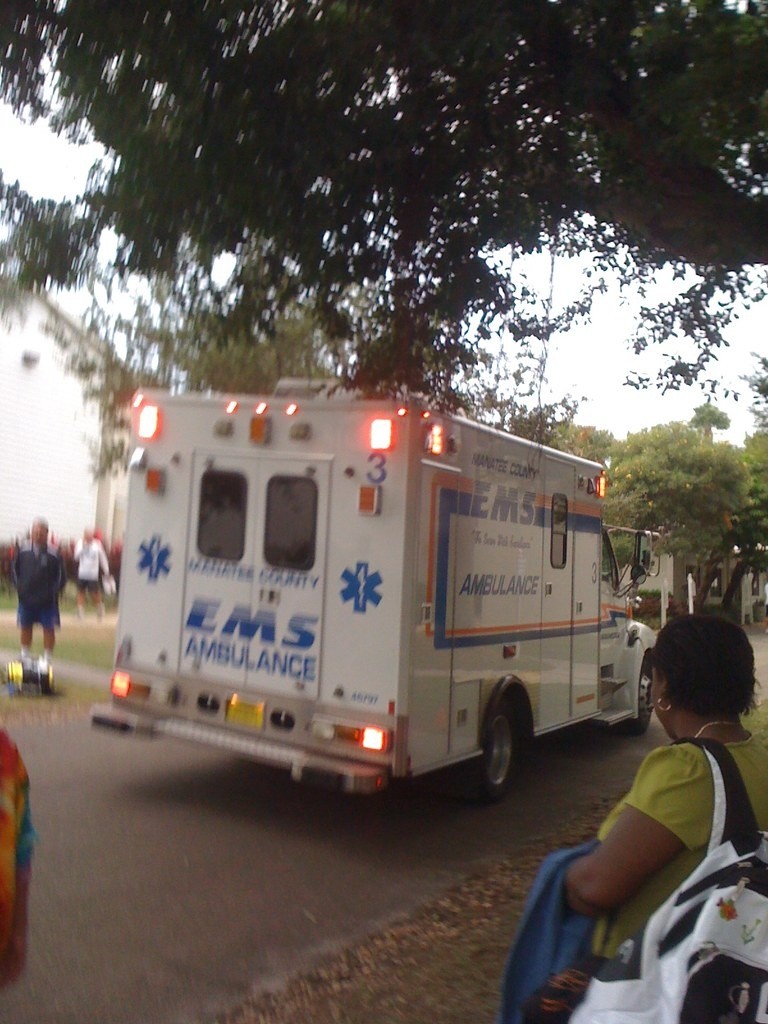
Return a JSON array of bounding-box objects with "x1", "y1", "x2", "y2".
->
[{"x1": 567, "y1": 736, "x2": 768, "y2": 1024}]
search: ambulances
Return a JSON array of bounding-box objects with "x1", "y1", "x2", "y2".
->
[{"x1": 90, "y1": 373, "x2": 660, "y2": 803}]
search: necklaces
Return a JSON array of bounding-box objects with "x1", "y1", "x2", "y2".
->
[{"x1": 694, "y1": 721, "x2": 741, "y2": 738}]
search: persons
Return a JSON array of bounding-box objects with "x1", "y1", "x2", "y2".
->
[
  {"x1": 12, "y1": 517, "x2": 67, "y2": 662},
  {"x1": 0, "y1": 724, "x2": 32, "y2": 994},
  {"x1": 73, "y1": 528, "x2": 110, "y2": 620},
  {"x1": 562, "y1": 614, "x2": 768, "y2": 1024}
]
